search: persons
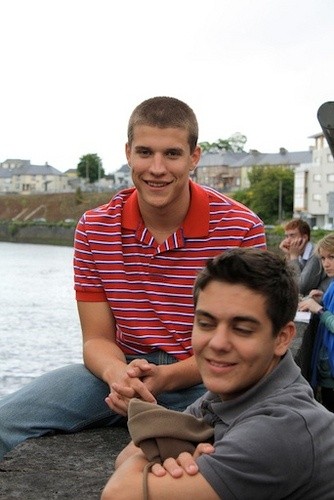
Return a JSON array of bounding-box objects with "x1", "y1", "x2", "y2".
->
[
  {"x1": 1, "y1": 95, "x2": 270, "y2": 457},
  {"x1": 99, "y1": 245, "x2": 334, "y2": 500},
  {"x1": 278, "y1": 217, "x2": 333, "y2": 300},
  {"x1": 297, "y1": 230, "x2": 334, "y2": 414}
]
